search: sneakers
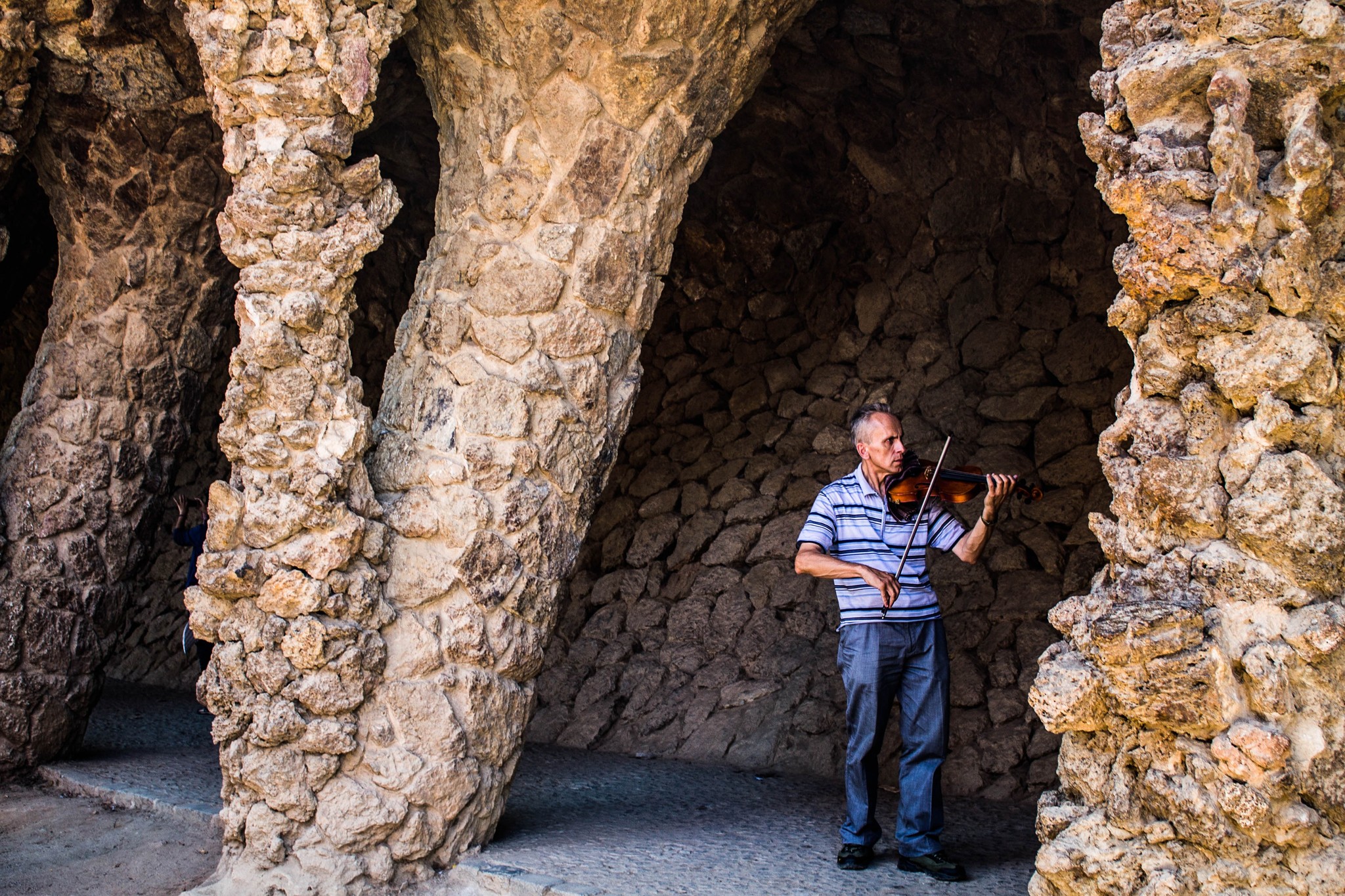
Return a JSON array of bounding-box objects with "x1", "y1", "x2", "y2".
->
[
  {"x1": 837, "y1": 843, "x2": 873, "y2": 870},
  {"x1": 897, "y1": 849, "x2": 965, "y2": 882}
]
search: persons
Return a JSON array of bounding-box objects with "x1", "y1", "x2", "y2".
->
[
  {"x1": 793, "y1": 402, "x2": 1019, "y2": 877},
  {"x1": 168, "y1": 490, "x2": 215, "y2": 718}
]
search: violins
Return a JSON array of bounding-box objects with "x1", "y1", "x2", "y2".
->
[{"x1": 887, "y1": 458, "x2": 1043, "y2": 504}]
